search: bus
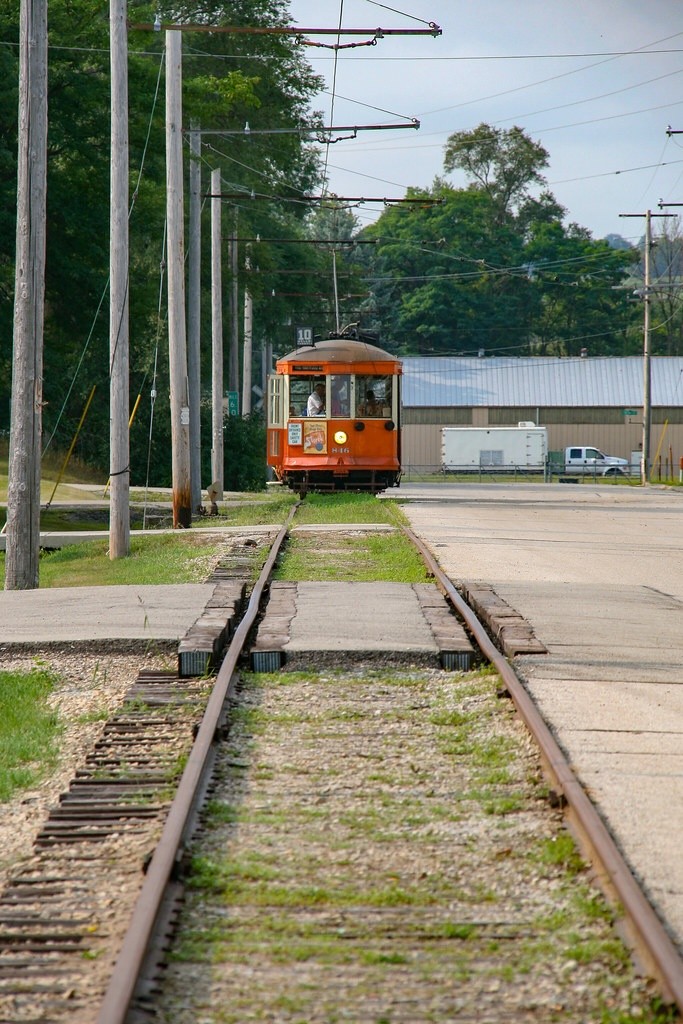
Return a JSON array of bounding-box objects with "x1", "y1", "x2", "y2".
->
[{"x1": 265, "y1": 338, "x2": 403, "y2": 496}]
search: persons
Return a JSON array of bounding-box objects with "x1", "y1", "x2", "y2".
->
[
  {"x1": 331, "y1": 378, "x2": 345, "y2": 415},
  {"x1": 307, "y1": 384, "x2": 325, "y2": 417}
]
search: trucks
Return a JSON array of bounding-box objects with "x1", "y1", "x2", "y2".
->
[{"x1": 440, "y1": 424, "x2": 630, "y2": 478}]
